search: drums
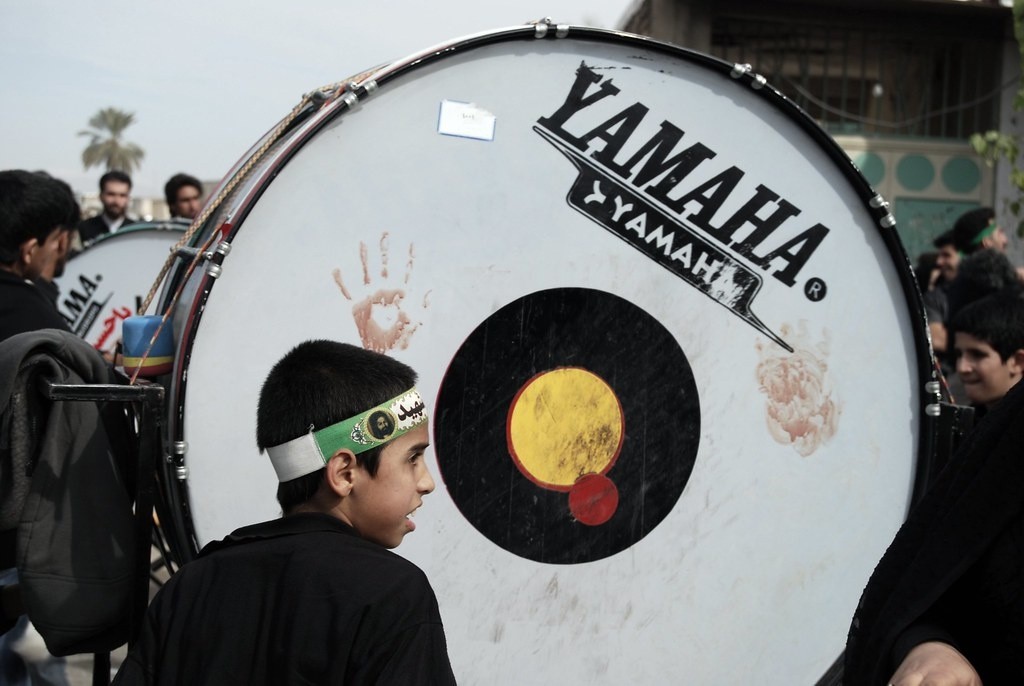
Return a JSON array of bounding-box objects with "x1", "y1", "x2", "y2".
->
[
  {"x1": 146, "y1": 19, "x2": 938, "y2": 686},
  {"x1": 28, "y1": 217, "x2": 197, "y2": 374}
]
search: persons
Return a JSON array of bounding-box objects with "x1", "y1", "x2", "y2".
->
[
  {"x1": 0, "y1": 164, "x2": 83, "y2": 686},
  {"x1": 844, "y1": 206, "x2": 1024, "y2": 686},
  {"x1": 163, "y1": 178, "x2": 203, "y2": 218},
  {"x1": 77, "y1": 172, "x2": 138, "y2": 242},
  {"x1": 111, "y1": 340, "x2": 460, "y2": 685}
]
